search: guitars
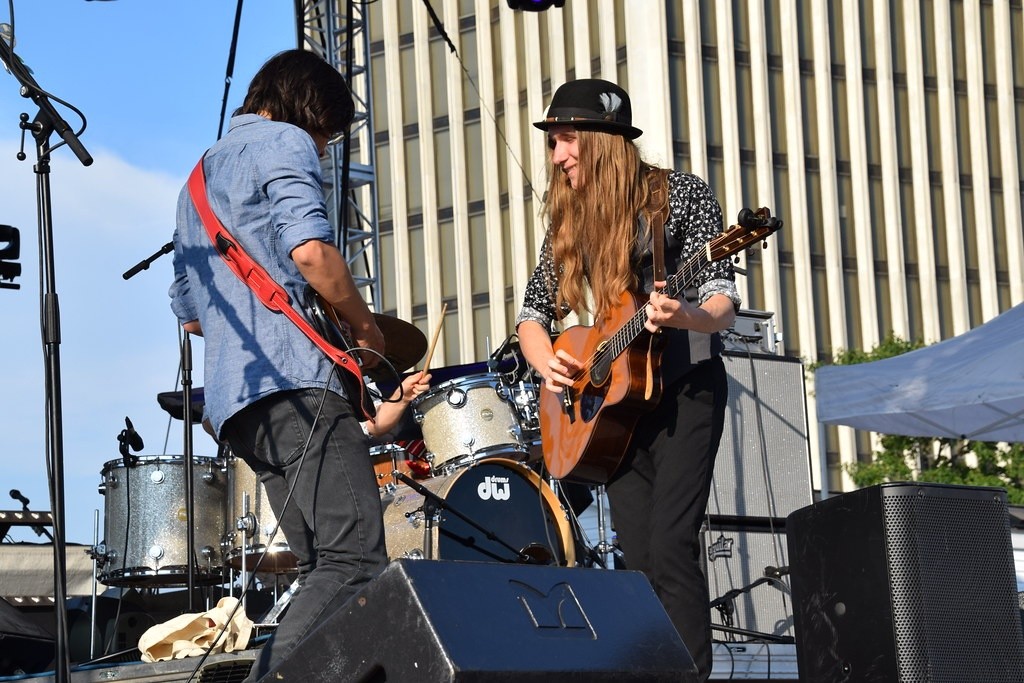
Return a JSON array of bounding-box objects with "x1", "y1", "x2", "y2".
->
[
  {"x1": 538, "y1": 207, "x2": 783, "y2": 487},
  {"x1": 303, "y1": 281, "x2": 377, "y2": 422}
]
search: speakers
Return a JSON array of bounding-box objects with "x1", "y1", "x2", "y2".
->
[
  {"x1": 697, "y1": 347, "x2": 814, "y2": 644},
  {"x1": 253, "y1": 561, "x2": 700, "y2": 683},
  {"x1": 787, "y1": 482, "x2": 1023, "y2": 683}
]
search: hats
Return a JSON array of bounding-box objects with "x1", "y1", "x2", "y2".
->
[{"x1": 532, "y1": 79, "x2": 643, "y2": 139}]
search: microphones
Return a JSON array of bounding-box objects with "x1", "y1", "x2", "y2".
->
[
  {"x1": 516, "y1": 543, "x2": 550, "y2": 566},
  {"x1": 487, "y1": 335, "x2": 513, "y2": 369},
  {"x1": 126, "y1": 416, "x2": 144, "y2": 451},
  {"x1": 764, "y1": 565, "x2": 789, "y2": 586}
]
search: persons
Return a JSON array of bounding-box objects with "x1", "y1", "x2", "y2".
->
[
  {"x1": 168, "y1": 47, "x2": 432, "y2": 683},
  {"x1": 515, "y1": 77, "x2": 742, "y2": 683}
]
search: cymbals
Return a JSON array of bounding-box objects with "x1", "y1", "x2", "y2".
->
[{"x1": 366, "y1": 310, "x2": 427, "y2": 373}]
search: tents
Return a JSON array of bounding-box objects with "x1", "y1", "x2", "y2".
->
[{"x1": 814, "y1": 301, "x2": 1023, "y2": 443}]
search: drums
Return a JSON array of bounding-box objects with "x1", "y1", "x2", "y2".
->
[
  {"x1": 223, "y1": 442, "x2": 300, "y2": 572},
  {"x1": 97, "y1": 454, "x2": 235, "y2": 589},
  {"x1": 381, "y1": 459, "x2": 578, "y2": 567},
  {"x1": 409, "y1": 371, "x2": 544, "y2": 470}
]
